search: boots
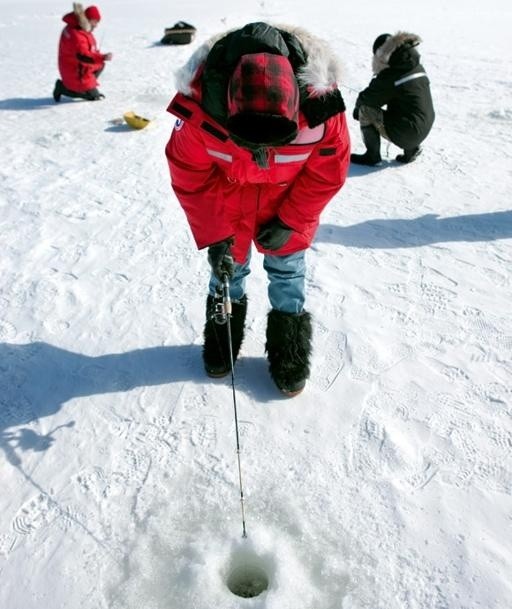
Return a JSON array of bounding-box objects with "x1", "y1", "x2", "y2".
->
[
  {"x1": 202, "y1": 295, "x2": 248, "y2": 381},
  {"x1": 348, "y1": 123, "x2": 385, "y2": 169}
]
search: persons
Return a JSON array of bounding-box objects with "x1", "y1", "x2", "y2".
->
[
  {"x1": 165, "y1": 21, "x2": 351, "y2": 397},
  {"x1": 347, "y1": 31, "x2": 436, "y2": 167},
  {"x1": 53, "y1": 2, "x2": 112, "y2": 102}
]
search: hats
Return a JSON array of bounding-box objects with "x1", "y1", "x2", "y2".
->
[{"x1": 84, "y1": 6, "x2": 101, "y2": 22}]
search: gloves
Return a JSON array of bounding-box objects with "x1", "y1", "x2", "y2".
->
[
  {"x1": 206, "y1": 241, "x2": 236, "y2": 283},
  {"x1": 254, "y1": 216, "x2": 293, "y2": 253}
]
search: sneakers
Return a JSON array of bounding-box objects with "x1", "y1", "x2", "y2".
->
[
  {"x1": 52, "y1": 79, "x2": 65, "y2": 103},
  {"x1": 394, "y1": 145, "x2": 423, "y2": 165}
]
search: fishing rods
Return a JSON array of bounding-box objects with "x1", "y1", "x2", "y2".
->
[{"x1": 212, "y1": 275, "x2": 249, "y2": 539}]
